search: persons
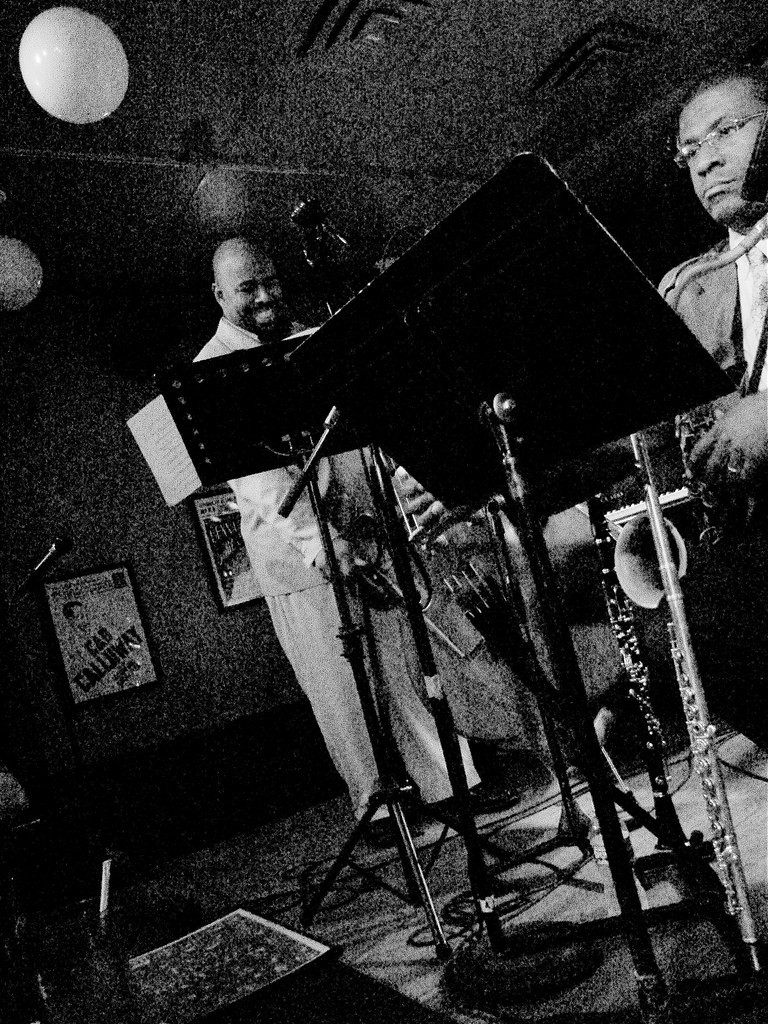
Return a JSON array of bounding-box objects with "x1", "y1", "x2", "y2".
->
[
  {"x1": 193, "y1": 238, "x2": 532, "y2": 847},
  {"x1": 395, "y1": 72, "x2": 766, "y2": 532}
]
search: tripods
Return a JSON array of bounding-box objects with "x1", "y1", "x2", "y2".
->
[{"x1": 150, "y1": 334, "x2": 650, "y2": 960}]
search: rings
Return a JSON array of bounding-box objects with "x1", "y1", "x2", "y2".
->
[{"x1": 728, "y1": 466, "x2": 741, "y2": 475}]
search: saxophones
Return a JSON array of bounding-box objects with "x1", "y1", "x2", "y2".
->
[{"x1": 611, "y1": 215, "x2": 768, "y2": 678}]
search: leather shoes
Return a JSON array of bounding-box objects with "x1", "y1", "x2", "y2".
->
[
  {"x1": 469, "y1": 786, "x2": 521, "y2": 815},
  {"x1": 371, "y1": 821, "x2": 418, "y2": 851}
]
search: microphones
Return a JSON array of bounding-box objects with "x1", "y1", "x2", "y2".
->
[{"x1": 17, "y1": 537, "x2": 74, "y2": 592}]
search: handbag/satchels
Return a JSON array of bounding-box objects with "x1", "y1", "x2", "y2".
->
[{"x1": 672, "y1": 526, "x2": 768, "y2": 753}]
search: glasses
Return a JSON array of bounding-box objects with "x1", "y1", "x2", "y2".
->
[{"x1": 674, "y1": 112, "x2": 768, "y2": 168}]
